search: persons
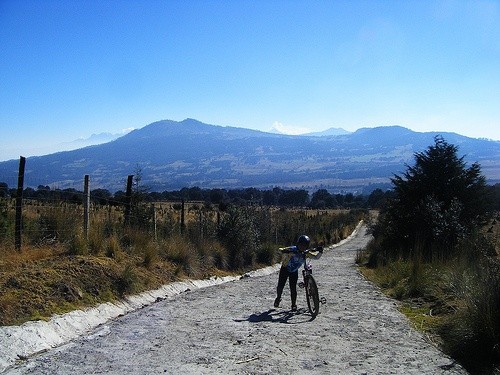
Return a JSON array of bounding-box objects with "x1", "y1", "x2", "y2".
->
[{"x1": 273, "y1": 234, "x2": 324, "y2": 311}]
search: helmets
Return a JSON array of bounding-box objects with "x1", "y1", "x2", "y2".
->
[{"x1": 296, "y1": 235, "x2": 310, "y2": 252}]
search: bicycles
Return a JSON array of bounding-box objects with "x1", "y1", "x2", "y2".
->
[{"x1": 282, "y1": 246, "x2": 327, "y2": 318}]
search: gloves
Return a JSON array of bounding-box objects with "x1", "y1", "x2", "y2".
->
[{"x1": 316, "y1": 247, "x2": 323, "y2": 253}]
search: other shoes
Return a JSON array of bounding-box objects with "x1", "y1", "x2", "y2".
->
[
  {"x1": 274, "y1": 298, "x2": 280, "y2": 307},
  {"x1": 292, "y1": 305, "x2": 297, "y2": 311}
]
